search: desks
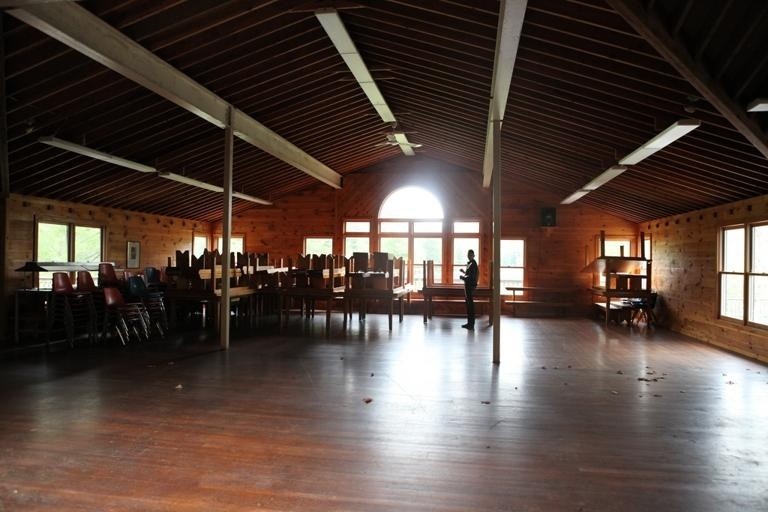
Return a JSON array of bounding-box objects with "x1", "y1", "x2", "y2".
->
[
  {"x1": 505, "y1": 287, "x2": 579, "y2": 316},
  {"x1": 420, "y1": 259, "x2": 495, "y2": 327},
  {"x1": 581, "y1": 228, "x2": 659, "y2": 330}
]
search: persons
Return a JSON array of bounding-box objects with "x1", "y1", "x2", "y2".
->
[{"x1": 459, "y1": 249, "x2": 478, "y2": 328}]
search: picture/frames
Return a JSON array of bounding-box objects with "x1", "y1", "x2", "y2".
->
[{"x1": 125, "y1": 240, "x2": 141, "y2": 269}]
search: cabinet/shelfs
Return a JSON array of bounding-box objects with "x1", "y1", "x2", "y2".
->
[{"x1": 7, "y1": 288, "x2": 52, "y2": 352}]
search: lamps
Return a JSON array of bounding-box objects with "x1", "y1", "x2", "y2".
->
[{"x1": 13, "y1": 262, "x2": 49, "y2": 292}]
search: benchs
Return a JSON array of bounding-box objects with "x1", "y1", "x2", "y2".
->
[
  {"x1": 503, "y1": 299, "x2": 573, "y2": 318},
  {"x1": 411, "y1": 299, "x2": 490, "y2": 315}
]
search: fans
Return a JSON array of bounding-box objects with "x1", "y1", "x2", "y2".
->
[{"x1": 375, "y1": 122, "x2": 424, "y2": 149}]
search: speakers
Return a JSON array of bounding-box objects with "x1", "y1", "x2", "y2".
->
[{"x1": 541, "y1": 207, "x2": 557, "y2": 226}]
